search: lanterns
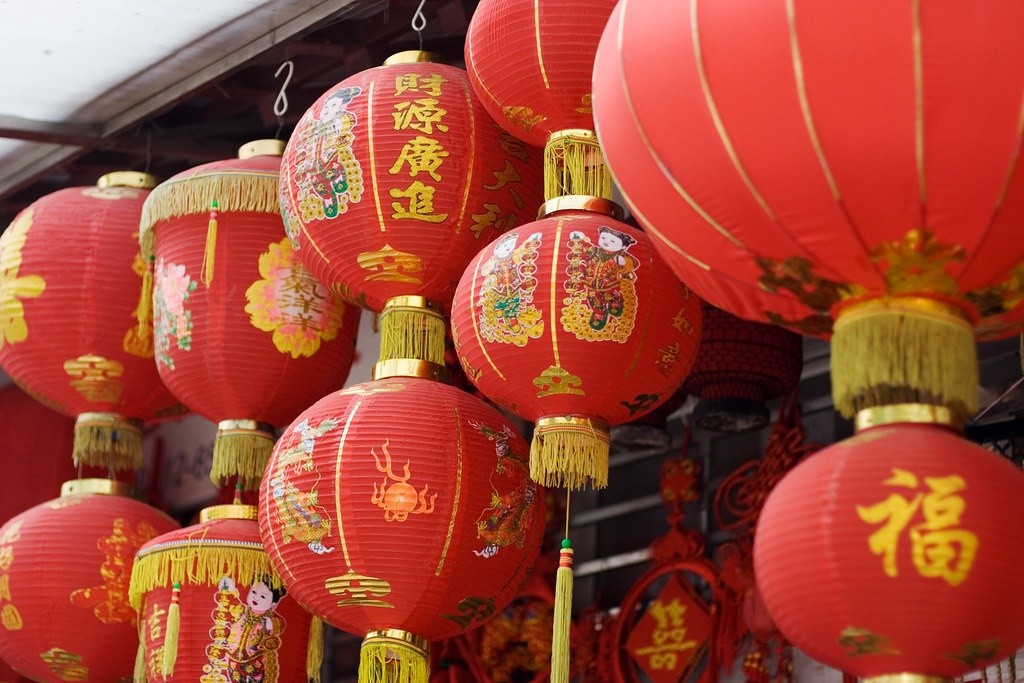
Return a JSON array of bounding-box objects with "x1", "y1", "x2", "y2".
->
[{"x1": 0, "y1": 0, "x2": 1024, "y2": 683}]
